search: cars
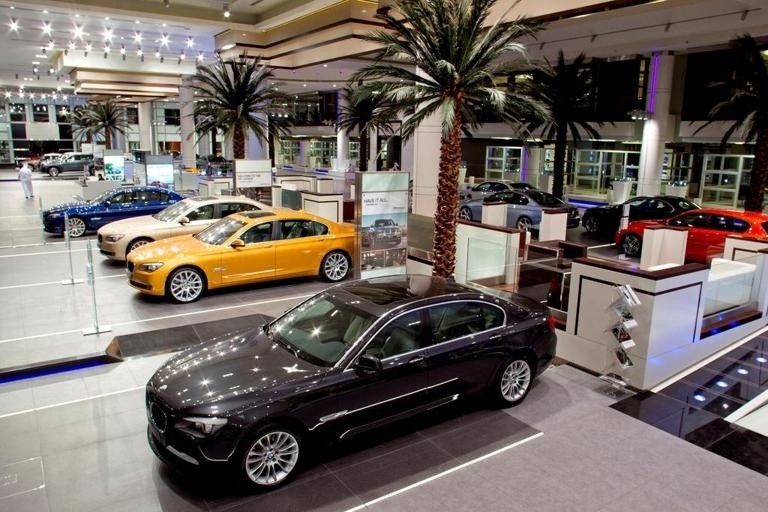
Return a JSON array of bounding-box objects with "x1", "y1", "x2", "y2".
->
[
  {"x1": 91, "y1": 192, "x2": 290, "y2": 267},
  {"x1": 124, "y1": 206, "x2": 361, "y2": 306},
  {"x1": 461, "y1": 178, "x2": 535, "y2": 201},
  {"x1": 163, "y1": 147, "x2": 233, "y2": 173},
  {"x1": 458, "y1": 187, "x2": 582, "y2": 239},
  {"x1": 139, "y1": 270, "x2": 561, "y2": 494},
  {"x1": 36, "y1": 182, "x2": 190, "y2": 241},
  {"x1": 15, "y1": 151, "x2": 62, "y2": 173},
  {"x1": 579, "y1": 193, "x2": 702, "y2": 238},
  {"x1": 616, "y1": 205, "x2": 768, "y2": 267}
]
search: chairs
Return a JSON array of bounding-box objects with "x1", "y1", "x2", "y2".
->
[
  {"x1": 527, "y1": 237, "x2": 589, "y2": 271},
  {"x1": 380, "y1": 315, "x2": 421, "y2": 357},
  {"x1": 324, "y1": 314, "x2": 374, "y2": 351}
]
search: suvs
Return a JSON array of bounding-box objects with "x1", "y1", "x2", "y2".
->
[
  {"x1": 36, "y1": 150, "x2": 96, "y2": 177},
  {"x1": 361, "y1": 225, "x2": 370, "y2": 246},
  {"x1": 366, "y1": 217, "x2": 402, "y2": 249}
]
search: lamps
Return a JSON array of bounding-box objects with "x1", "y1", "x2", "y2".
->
[{"x1": 0, "y1": 1, "x2": 232, "y2": 100}]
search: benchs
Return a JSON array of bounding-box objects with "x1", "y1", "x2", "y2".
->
[{"x1": 647, "y1": 256, "x2": 756, "y2": 318}]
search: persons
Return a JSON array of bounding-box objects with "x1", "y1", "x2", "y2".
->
[
  {"x1": 18, "y1": 161, "x2": 34, "y2": 200},
  {"x1": 98, "y1": 174, "x2": 105, "y2": 180}
]
